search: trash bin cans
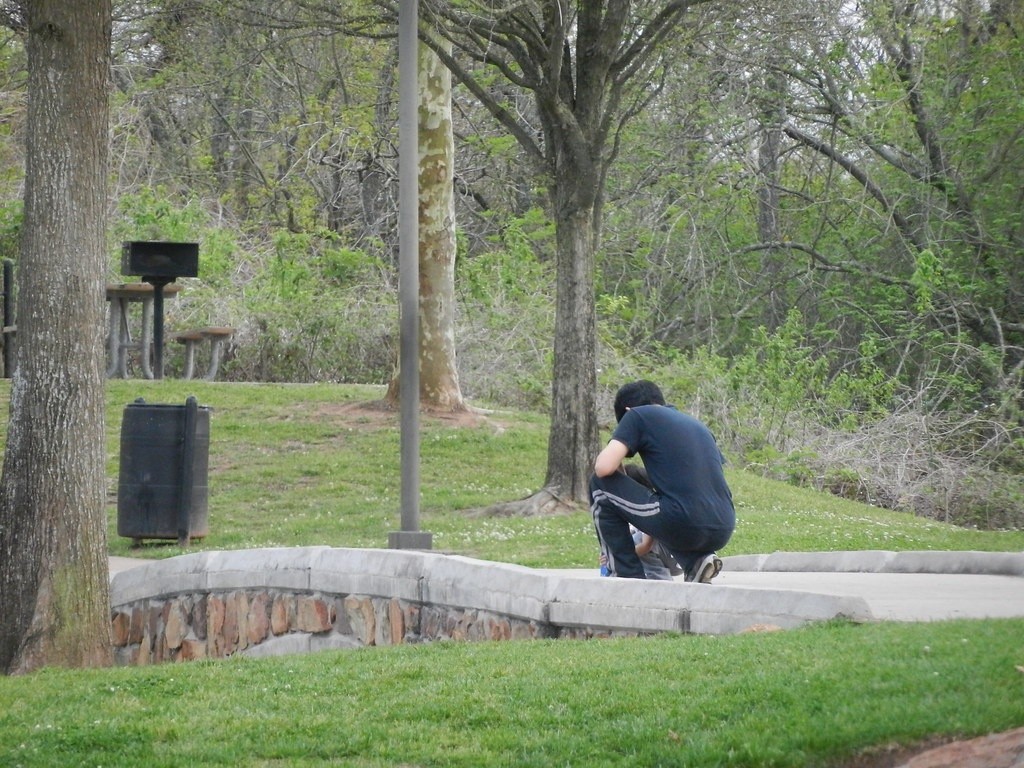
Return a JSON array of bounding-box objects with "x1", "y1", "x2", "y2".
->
[{"x1": 116, "y1": 395, "x2": 213, "y2": 550}]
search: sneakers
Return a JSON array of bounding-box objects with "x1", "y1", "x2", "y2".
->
[{"x1": 683, "y1": 552, "x2": 723, "y2": 583}]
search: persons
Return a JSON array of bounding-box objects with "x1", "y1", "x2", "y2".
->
[{"x1": 587, "y1": 380, "x2": 736, "y2": 584}]
